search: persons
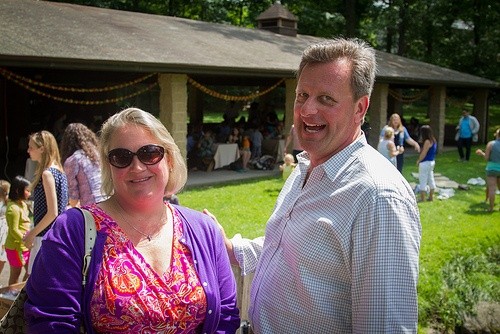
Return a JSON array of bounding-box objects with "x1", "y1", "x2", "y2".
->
[
  {"x1": 24, "y1": 108, "x2": 241, "y2": 334},
  {"x1": 476, "y1": 129, "x2": 500, "y2": 211},
  {"x1": 456, "y1": 109, "x2": 480, "y2": 161},
  {"x1": 378, "y1": 114, "x2": 437, "y2": 201},
  {"x1": 204, "y1": 40, "x2": 422, "y2": 334},
  {"x1": 188, "y1": 122, "x2": 304, "y2": 181},
  {"x1": 25, "y1": 131, "x2": 68, "y2": 274},
  {"x1": 0, "y1": 176, "x2": 33, "y2": 285},
  {"x1": 64, "y1": 123, "x2": 111, "y2": 208}
]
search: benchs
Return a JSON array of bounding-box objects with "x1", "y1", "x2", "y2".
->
[{"x1": 203, "y1": 156, "x2": 214, "y2": 160}]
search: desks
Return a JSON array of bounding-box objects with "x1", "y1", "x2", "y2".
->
[
  {"x1": 207, "y1": 142, "x2": 241, "y2": 172},
  {"x1": 261, "y1": 139, "x2": 286, "y2": 164}
]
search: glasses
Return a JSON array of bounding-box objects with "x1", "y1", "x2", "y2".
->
[{"x1": 105, "y1": 144, "x2": 165, "y2": 169}]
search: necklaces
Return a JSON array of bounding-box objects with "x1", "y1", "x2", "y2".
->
[{"x1": 112, "y1": 196, "x2": 164, "y2": 242}]
